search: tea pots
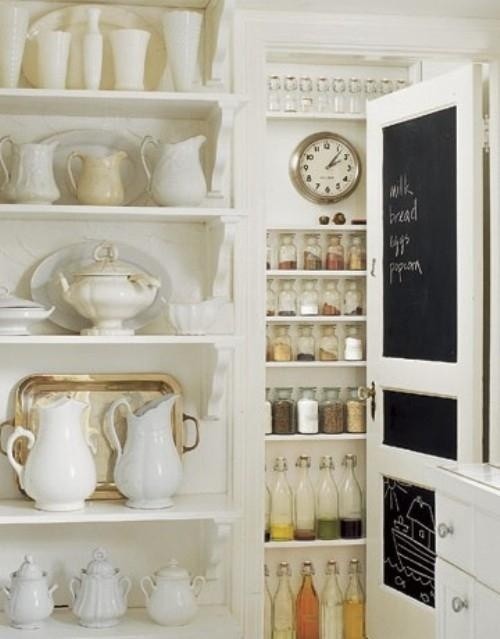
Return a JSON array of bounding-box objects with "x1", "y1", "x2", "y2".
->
[
  {"x1": 57, "y1": 239, "x2": 162, "y2": 337},
  {"x1": 1, "y1": 286, "x2": 55, "y2": 336},
  {"x1": 66, "y1": 150, "x2": 128, "y2": 205},
  {"x1": 140, "y1": 134, "x2": 206, "y2": 207},
  {"x1": 108, "y1": 394, "x2": 186, "y2": 510},
  {"x1": 2, "y1": 554, "x2": 58, "y2": 631},
  {"x1": 7, "y1": 397, "x2": 98, "y2": 511},
  {"x1": 139, "y1": 560, "x2": 206, "y2": 627},
  {"x1": 1, "y1": 134, "x2": 61, "y2": 204},
  {"x1": 69, "y1": 548, "x2": 132, "y2": 630}
]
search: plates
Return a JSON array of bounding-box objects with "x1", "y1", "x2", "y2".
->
[
  {"x1": 0, "y1": 373, "x2": 200, "y2": 500},
  {"x1": 28, "y1": 127, "x2": 158, "y2": 203},
  {"x1": 21, "y1": 3, "x2": 166, "y2": 92},
  {"x1": 29, "y1": 241, "x2": 175, "y2": 337}
]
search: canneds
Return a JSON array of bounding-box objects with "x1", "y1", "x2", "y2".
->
[{"x1": 346, "y1": 386, "x2": 366, "y2": 434}]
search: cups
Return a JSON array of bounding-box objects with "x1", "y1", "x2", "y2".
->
[
  {"x1": 164, "y1": 10, "x2": 203, "y2": 93},
  {"x1": 0, "y1": 7, "x2": 30, "y2": 87},
  {"x1": 109, "y1": 28, "x2": 151, "y2": 91},
  {"x1": 35, "y1": 30, "x2": 70, "y2": 89}
]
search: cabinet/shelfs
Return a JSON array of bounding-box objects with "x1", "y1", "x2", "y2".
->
[
  {"x1": 432, "y1": 463, "x2": 499, "y2": 637},
  {"x1": 264, "y1": 227, "x2": 366, "y2": 638},
  {"x1": 0, "y1": 1, "x2": 242, "y2": 634}
]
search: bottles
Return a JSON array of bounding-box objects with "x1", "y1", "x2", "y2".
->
[
  {"x1": 272, "y1": 386, "x2": 296, "y2": 434},
  {"x1": 319, "y1": 278, "x2": 342, "y2": 315},
  {"x1": 345, "y1": 233, "x2": 366, "y2": 270},
  {"x1": 265, "y1": 279, "x2": 276, "y2": 317},
  {"x1": 325, "y1": 233, "x2": 345, "y2": 270},
  {"x1": 277, "y1": 278, "x2": 298, "y2": 317},
  {"x1": 318, "y1": 324, "x2": 339, "y2": 361},
  {"x1": 277, "y1": 233, "x2": 297, "y2": 270},
  {"x1": 270, "y1": 456, "x2": 293, "y2": 541},
  {"x1": 318, "y1": 386, "x2": 345, "y2": 434},
  {"x1": 319, "y1": 559, "x2": 343, "y2": 639},
  {"x1": 272, "y1": 325, "x2": 293, "y2": 361},
  {"x1": 266, "y1": 232, "x2": 271, "y2": 269},
  {"x1": 293, "y1": 455, "x2": 316, "y2": 540},
  {"x1": 272, "y1": 561, "x2": 295, "y2": 639},
  {"x1": 296, "y1": 386, "x2": 319, "y2": 434},
  {"x1": 265, "y1": 387, "x2": 272, "y2": 435},
  {"x1": 338, "y1": 453, "x2": 362, "y2": 539},
  {"x1": 264, "y1": 465, "x2": 270, "y2": 543},
  {"x1": 295, "y1": 560, "x2": 320, "y2": 639},
  {"x1": 303, "y1": 232, "x2": 322, "y2": 270},
  {"x1": 263, "y1": 563, "x2": 273, "y2": 639},
  {"x1": 343, "y1": 324, "x2": 363, "y2": 361},
  {"x1": 343, "y1": 558, "x2": 365, "y2": 639},
  {"x1": 296, "y1": 324, "x2": 315, "y2": 362},
  {"x1": 316, "y1": 455, "x2": 339, "y2": 540},
  {"x1": 342, "y1": 278, "x2": 364, "y2": 315},
  {"x1": 299, "y1": 279, "x2": 319, "y2": 316}
]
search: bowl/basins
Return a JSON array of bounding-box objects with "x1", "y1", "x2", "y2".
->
[{"x1": 167, "y1": 296, "x2": 226, "y2": 335}]
